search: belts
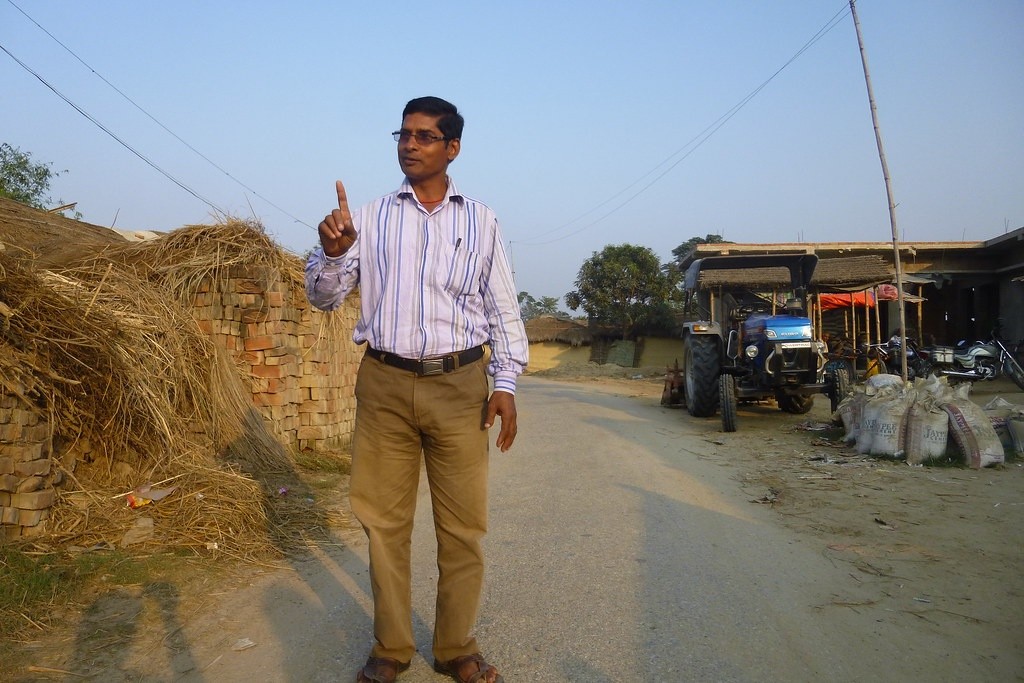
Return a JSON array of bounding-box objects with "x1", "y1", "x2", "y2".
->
[{"x1": 367, "y1": 346, "x2": 484, "y2": 376}]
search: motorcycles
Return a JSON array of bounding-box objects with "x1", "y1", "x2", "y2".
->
[
  {"x1": 919, "y1": 318, "x2": 1024, "y2": 391},
  {"x1": 868, "y1": 331, "x2": 933, "y2": 383}
]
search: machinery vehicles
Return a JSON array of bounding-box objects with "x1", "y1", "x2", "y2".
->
[{"x1": 660, "y1": 252, "x2": 851, "y2": 431}]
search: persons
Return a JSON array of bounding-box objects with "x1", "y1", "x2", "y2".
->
[
  {"x1": 856, "y1": 333, "x2": 867, "y2": 348},
  {"x1": 866, "y1": 348, "x2": 879, "y2": 379},
  {"x1": 822, "y1": 331, "x2": 830, "y2": 354},
  {"x1": 304, "y1": 95, "x2": 530, "y2": 683}
]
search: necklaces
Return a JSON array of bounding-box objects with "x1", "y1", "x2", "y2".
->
[{"x1": 420, "y1": 198, "x2": 444, "y2": 203}]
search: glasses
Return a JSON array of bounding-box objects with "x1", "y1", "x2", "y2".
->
[{"x1": 392, "y1": 131, "x2": 449, "y2": 146}]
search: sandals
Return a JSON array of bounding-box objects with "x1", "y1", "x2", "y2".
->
[
  {"x1": 434, "y1": 653, "x2": 502, "y2": 683},
  {"x1": 357, "y1": 656, "x2": 411, "y2": 683}
]
